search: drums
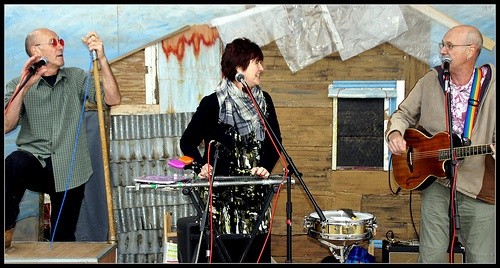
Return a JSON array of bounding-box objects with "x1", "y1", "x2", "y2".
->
[{"x1": 306, "y1": 210, "x2": 376, "y2": 241}]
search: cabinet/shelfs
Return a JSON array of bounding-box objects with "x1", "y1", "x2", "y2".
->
[{"x1": 5, "y1": 241, "x2": 118, "y2": 263}]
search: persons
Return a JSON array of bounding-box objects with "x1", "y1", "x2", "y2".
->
[
  {"x1": 179, "y1": 38, "x2": 282, "y2": 178},
  {"x1": 385, "y1": 25, "x2": 496, "y2": 263},
  {"x1": 4, "y1": 28, "x2": 121, "y2": 248}
]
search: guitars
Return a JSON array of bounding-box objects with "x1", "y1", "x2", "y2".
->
[{"x1": 392, "y1": 125, "x2": 496, "y2": 192}]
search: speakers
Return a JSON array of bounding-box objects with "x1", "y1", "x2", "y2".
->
[{"x1": 381, "y1": 240, "x2": 465, "y2": 264}]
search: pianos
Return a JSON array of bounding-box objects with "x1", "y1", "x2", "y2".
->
[{"x1": 122, "y1": 166, "x2": 297, "y2": 190}]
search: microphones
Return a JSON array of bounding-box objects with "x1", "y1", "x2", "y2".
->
[
  {"x1": 28, "y1": 57, "x2": 48, "y2": 72},
  {"x1": 442, "y1": 55, "x2": 453, "y2": 76},
  {"x1": 211, "y1": 140, "x2": 231, "y2": 156},
  {"x1": 235, "y1": 73, "x2": 248, "y2": 87}
]
